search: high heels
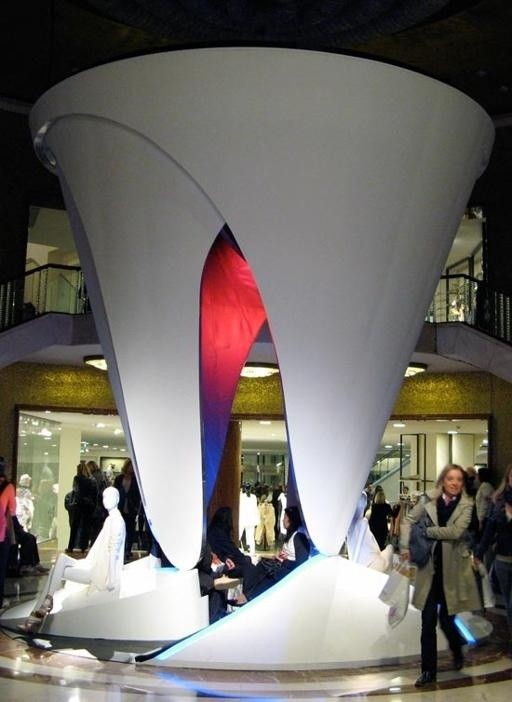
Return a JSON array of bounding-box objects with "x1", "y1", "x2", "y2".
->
[{"x1": 17, "y1": 595, "x2": 53, "y2": 633}]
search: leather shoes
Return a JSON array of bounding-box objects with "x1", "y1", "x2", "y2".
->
[
  {"x1": 415, "y1": 671, "x2": 437, "y2": 686},
  {"x1": 456, "y1": 663, "x2": 462, "y2": 670},
  {"x1": 19, "y1": 563, "x2": 49, "y2": 577}
]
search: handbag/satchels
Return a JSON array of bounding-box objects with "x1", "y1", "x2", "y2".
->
[
  {"x1": 256, "y1": 557, "x2": 284, "y2": 578},
  {"x1": 409, "y1": 516, "x2": 431, "y2": 568},
  {"x1": 8, "y1": 516, "x2": 16, "y2": 545}
]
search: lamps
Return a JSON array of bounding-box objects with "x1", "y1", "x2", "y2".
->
[
  {"x1": 240, "y1": 362, "x2": 279, "y2": 378},
  {"x1": 84, "y1": 355, "x2": 108, "y2": 371},
  {"x1": 403, "y1": 362, "x2": 427, "y2": 377}
]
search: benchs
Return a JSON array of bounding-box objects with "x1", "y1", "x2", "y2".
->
[{"x1": 213, "y1": 575, "x2": 245, "y2": 612}]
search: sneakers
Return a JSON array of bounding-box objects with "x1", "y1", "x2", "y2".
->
[{"x1": 213, "y1": 574, "x2": 240, "y2": 590}]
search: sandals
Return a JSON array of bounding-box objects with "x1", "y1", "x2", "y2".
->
[{"x1": 227, "y1": 600, "x2": 246, "y2": 606}]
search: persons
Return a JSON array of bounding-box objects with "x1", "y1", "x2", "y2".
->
[
  {"x1": 397, "y1": 462, "x2": 486, "y2": 690},
  {"x1": 362, "y1": 463, "x2": 512, "y2": 626},
  {"x1": 198, "y1": 481, "x2": 312, "y2": 627},
  {"x1": 1, "y1": 454, "x2": 150, "y2": 612}
]
